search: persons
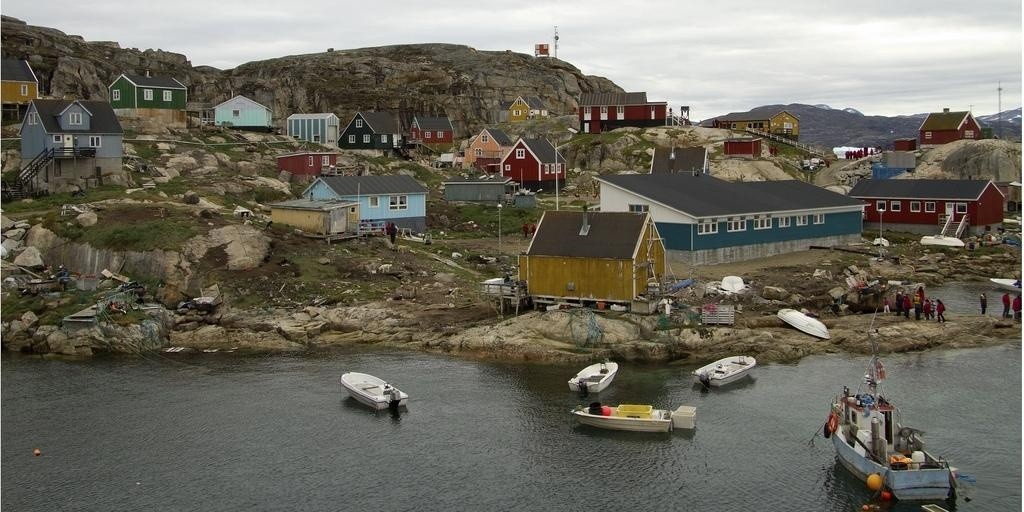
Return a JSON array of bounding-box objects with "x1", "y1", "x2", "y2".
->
[
  {"x1": 980, "y1": 293, "x2": 987, "y2": 314},
  {"x1": 389, "y1": 223, "x2": 397, "y2": 244},
  {"x1": 1012, "y1": 296, "x2": 1022, "y2": 313},
  {"x1": 1002, "y1": 292, "x2": 1010, "y2": 317},
  {"x1": 884, "y1": 286, "x2": 946, "y2": 323},
  {"x1": 59, "y1": 268, "x2": 69, "y2": 292},
  {"x1": 523, "y1": 224, "x2": 529, "y2": 238},
  {"x1": 531, "y1": 224, "x2": 536, "y2": 236},
  {"x1": 386, "y1": 223, "x2": 391, "y2": 235},
  {"x1": 845, "y1": 147, "x2": 874, "y2": 160}
]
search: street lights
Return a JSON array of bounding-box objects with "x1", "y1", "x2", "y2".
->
[
  {"x1": 496, "y1": 203, "x2": 503, "y2": 257},
  {"x1": 877, "y1": 208, "x2": 886, "y2": 262}
]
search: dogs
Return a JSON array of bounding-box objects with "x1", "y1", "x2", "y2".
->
[
  {"x1": 263, "y1": 221, "x2": 273, "y2": 231},
  {"x1": 379, "y1": 264, "x2": 393, "y2": 273}
]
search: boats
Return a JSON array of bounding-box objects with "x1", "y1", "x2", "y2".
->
[
  {"x1": 921, "y1": 234, "x2": 965, "y2": 249},
  {"x1": 567, "y1": 356, "x2": 619, "y2": 396},
  {"x1": 777, "y1": 307, "x2": 830, "y2": 341},
  {"x1": 825, "y1": 323, "x2": 960, "y2": 505},
  {"x1": 338, "y1": 369, "x2": 407, "y2": 411},
  {"x1": 568, "y1": 403, "x2": 697, "y2": 434},
  {"x1": 691, "y1": 351, "x2": 758, "y2": 388},
  {"x1": 987, "y1": 277, "x2": 1021, "y2": 293}
]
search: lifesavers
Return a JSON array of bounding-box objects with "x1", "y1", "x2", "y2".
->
[{"x1": 824, "y1": 411, "x2": 838, "y2": 438}]
